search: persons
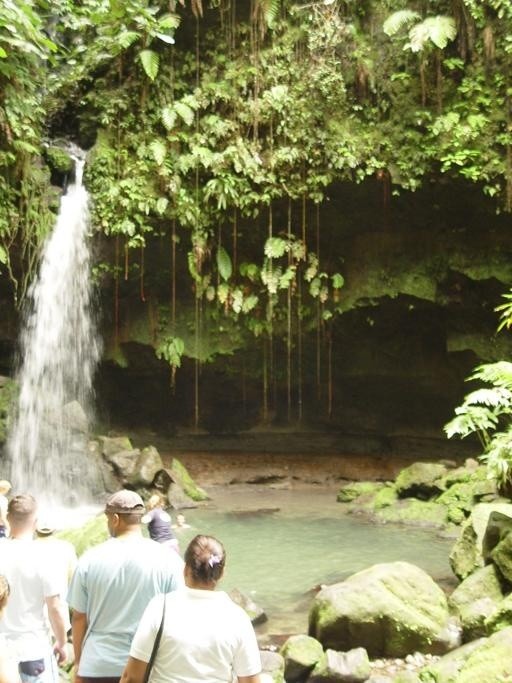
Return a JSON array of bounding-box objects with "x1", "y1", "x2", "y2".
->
[
  {"x1": 0, "y1": 479, "x2": 12, "y2": 540},
  {"x1": 171, "y1": 513, "x2": 195, "y2": 532},
  {"x1": 0, "y1": 573, "x2": 20, "y2": 683},
  {"x1": 140, "y1": 495, "x2": 179, "y2": 555},
  {"x1": 32, "y1": 504, "x2": 78, "y2": 637},
  {"x1": 66, "y1": 489, "x2": 186, "y2": 683},
  {"x1": 118, "y1": 536, "x2": 264, "y2": 683},
  {"x1": 0, "y1": 494, "x2": 68, "y2": 681}
]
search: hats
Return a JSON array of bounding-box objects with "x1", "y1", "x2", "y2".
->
[{"x1": 95, "y1": 489, "x2": 146, "y2": 518}]
